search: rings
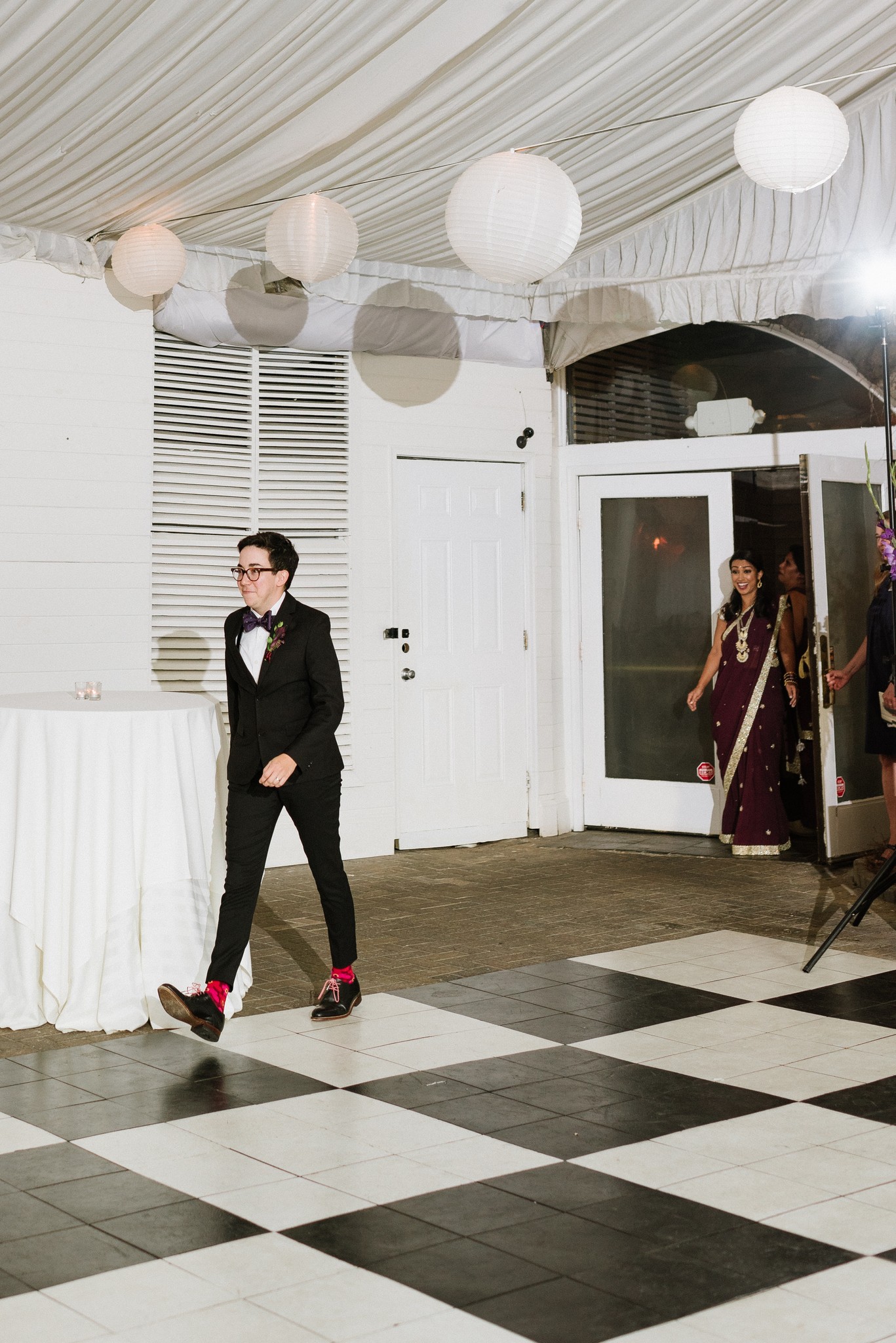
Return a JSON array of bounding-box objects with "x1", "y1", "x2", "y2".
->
[
  {"x1": 889, "y1": 704, "x2": 892, "y2": 705},
  {"x1": 276, "y1": 778, "x2": 280, "y2": 782}
]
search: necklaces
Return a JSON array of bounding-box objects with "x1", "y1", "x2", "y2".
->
[{"x1": 735, "y1": 609, "x2": 754, "y2": 663}]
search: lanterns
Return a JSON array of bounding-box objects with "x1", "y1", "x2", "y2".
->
[
  {"x1": 734, "y1": 86, "x2": 850, "y2": 193},
  {"x1": 265, "y1": 194, "x2": 359, "y2": 283},
  {"x1": 445, "y1": 152, "x2": 582, "y2": 286},
  {"x1": 111, "y1": 224, "x2": 186, "y2": 296}
]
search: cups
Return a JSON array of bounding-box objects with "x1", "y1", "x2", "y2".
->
[{"x1": 75, "y1": 681, "x2": 102, "y2": 700}]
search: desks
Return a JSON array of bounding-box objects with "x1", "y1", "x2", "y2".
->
[{"x1": 0, "y1": 688, "x2": 256, "y2": 1037}]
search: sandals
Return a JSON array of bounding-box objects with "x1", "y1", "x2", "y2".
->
[{"x1": 871, "y1": 844, "x2": 896, "y2": 867}]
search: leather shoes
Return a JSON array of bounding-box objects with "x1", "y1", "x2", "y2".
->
[
  {"x1": 158, "y1": 984, "x2": 226, "y2": 1042},
  {"x1": 311, "y1": 973, "x2": 362, "y2": 1021}
]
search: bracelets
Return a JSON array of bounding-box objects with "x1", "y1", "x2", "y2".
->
[{"x1": 784, "y1": 672, "x2": 798, "y2": 685}]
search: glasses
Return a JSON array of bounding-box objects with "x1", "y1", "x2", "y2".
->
[{"x1": 231, "y1": 567, "x2": 284, "y2": 582}]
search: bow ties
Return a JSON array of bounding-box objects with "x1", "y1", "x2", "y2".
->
[{"x1": 243, "y1": 611, "x2": 276, "y2": 633}]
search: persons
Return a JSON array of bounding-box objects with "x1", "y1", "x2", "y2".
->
[
  {"x1": 778, "y1": 548, "x2": 818, "y2": 831},
  {"x1": 157, "y1": 533, "x2": 362, "y2": 1042},
  {"x1": 827, "y1": 511, "x2": 896, "y2": 868},
  {"x1": 687, "y1": 551, "x2": 798, "y2": 855}
]
